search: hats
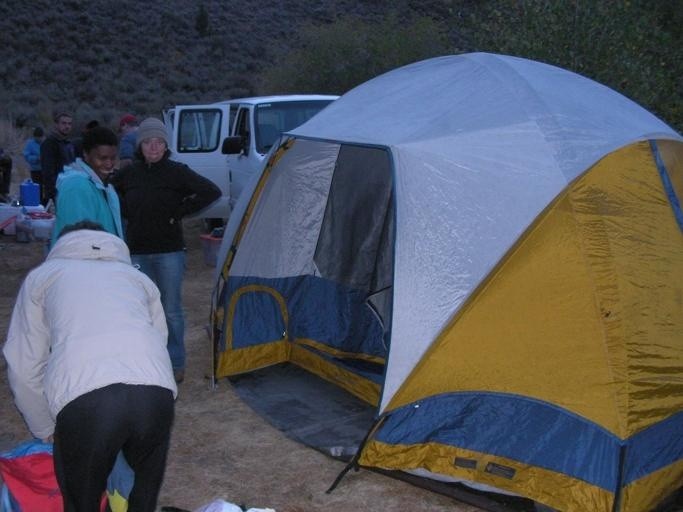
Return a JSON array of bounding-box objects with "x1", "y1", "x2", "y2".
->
[
  {"x1": 134, "y1": 116, "x2": 169, "y2": 151},
  {"x1": 116, "y1": 113, "x2": 137, "y2": 134},
  {"x1": 32, "y1": 126, "x2": 44, "y2": 137}
]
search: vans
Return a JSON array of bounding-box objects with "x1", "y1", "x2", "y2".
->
[{"x1": 161, "y1": 94, "x2": 340, "y2": 231}]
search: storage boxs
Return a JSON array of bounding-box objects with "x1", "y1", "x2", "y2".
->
[
  {"x1": 0, "y1": 183, "x2": 57, "y2": 241},
  {"x1": 199, "y1": 234, "x2": 223, "y2": 268}
]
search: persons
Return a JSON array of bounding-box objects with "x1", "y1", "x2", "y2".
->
[
  {"x1": 22, "y1": 126, "x2": 44, "y2": 203},
  {"x1": 3, "y1": 218, "x2": 177, "y2": 512},
  {"x1": 117, "y1": 115, "x2": 139, "y2": 168},
  {"x1": 48, "y1": 128, "x2": 122, "y2": 249},
  {"x1": 115, "y1": 118, "x2": 223, "y2": 382},
  {"x1": 37, "y1": 110, "x2": 76, "y2": 206}
]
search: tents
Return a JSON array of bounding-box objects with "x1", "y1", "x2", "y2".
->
[{"x1": 202, "y1": 51, "x2": 680, "y2": 512}]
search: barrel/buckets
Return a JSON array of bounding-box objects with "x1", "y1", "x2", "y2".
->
[{"x1": 19, "y1": 179, "x2": 40, "y2": 206}]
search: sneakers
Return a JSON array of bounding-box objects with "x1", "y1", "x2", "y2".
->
[{"x1": 171, "y1": 365, "x2": 187, "y2": 384}]
